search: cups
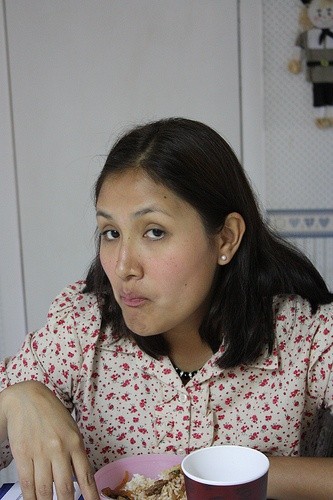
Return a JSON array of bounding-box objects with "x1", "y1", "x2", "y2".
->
[{"x1": 181, "y1": 445, "x2": 270, "y2": 500}]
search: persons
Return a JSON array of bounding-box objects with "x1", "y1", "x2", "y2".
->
[{"x1": 0, "y1": 118, "x2": 333, "y2": 500}]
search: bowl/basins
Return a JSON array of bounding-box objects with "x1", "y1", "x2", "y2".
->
[{"x1": 94, "y1": 454, "x2": 186, "y2": 500}]
